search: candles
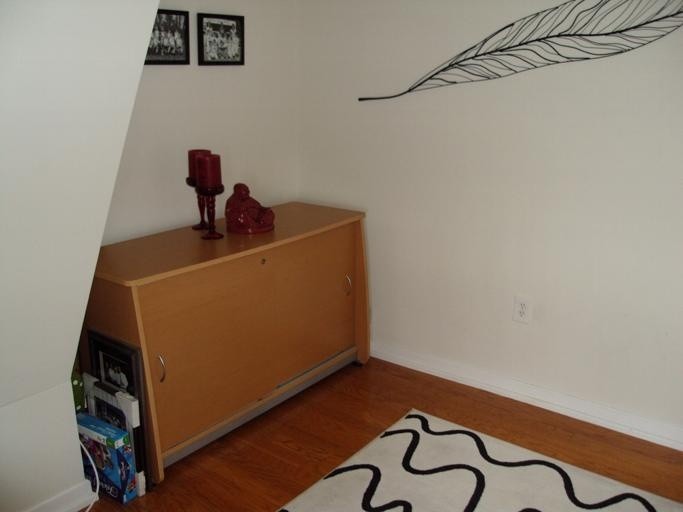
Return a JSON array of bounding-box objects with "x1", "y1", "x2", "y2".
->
[
  {"x1": 187, "y1": 148, "x2": 211, "y2": 176},
  {"x1": 196, "y1": 155, "x2": 223, "y2": 187}
]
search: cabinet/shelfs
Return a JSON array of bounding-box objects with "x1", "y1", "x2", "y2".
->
[{"x1": 90, "y1": 203, "x2": 371, "y2": 487}]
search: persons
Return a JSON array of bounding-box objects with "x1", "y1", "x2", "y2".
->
[
  {"x1": 224, "y1": 181, "x2": 273, "y2": 232},
  {"x1": 146, "y1": 15, "x2": 240, "y2": 61}
]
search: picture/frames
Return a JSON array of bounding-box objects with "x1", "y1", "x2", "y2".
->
[
  {"x1": 80, "y1": 371, "x2": 145, "y2": 497},
  {"x1": 195, "y1": 12, "x2": 245, "y2": 66},
  {"x1": 145, "y1": 9, "x2": 191, "y2": 67},
  {"x1": 86, "y1": 331, "x2": 141, "y2": 397}
]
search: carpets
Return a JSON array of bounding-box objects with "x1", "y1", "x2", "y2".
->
[{"x1": 264, "y1": 406, "x2": 682, "y2": 510}]
